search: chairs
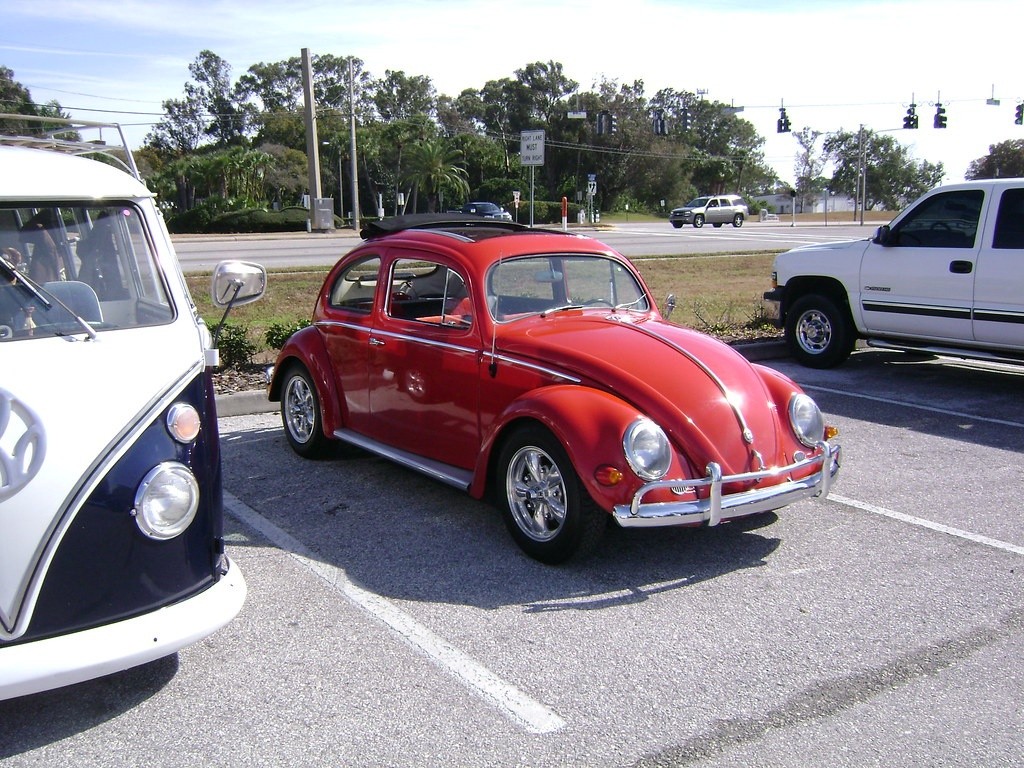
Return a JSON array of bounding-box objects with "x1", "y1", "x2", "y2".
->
[{"x1": 0, "y1": 281, "x2": 104, "y2": 329}]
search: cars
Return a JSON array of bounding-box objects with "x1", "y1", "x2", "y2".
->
[{"x1": 267, "y1": 213, "x2": 841, "y2": 569}]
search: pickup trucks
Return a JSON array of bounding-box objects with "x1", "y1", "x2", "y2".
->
[{"x1": 445, "y1": 202, "x2": 513, "y2": 222}]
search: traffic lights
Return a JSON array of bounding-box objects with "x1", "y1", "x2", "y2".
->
[
  {"x1": 934, "y1": 108, "x2": 947, "y2": 129},
  {"x1": 903, "y1": 107, "x2": 918, "y2": 129},
  {"x1": 1014, "y1": 103, "x2": 1024, "y2": 126},
  {"x1": 681, "y1": 110, "x2": 691, "y2": 134},
  {"x1": 602, "y1": 115, "x2": 617, "y2": 136},
  {"x1": 776, "y1": 112, "x2": 792, "y2": 133}
]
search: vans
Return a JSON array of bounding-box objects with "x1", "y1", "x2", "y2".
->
[{"x1": 2, "y1": 111, "x2": 266, "y2": 706}]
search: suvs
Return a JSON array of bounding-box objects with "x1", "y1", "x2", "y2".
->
[
  {"x1": 762, "y1": 176, "x2": 1024, "y2": 371},
  {"x1": 669, "y1": 194, "x2": 749, "y2": 229}
]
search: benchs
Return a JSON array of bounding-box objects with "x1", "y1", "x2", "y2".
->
[{"x1": 355, "y1": 297, "x2": 457, "y2": 321}]
